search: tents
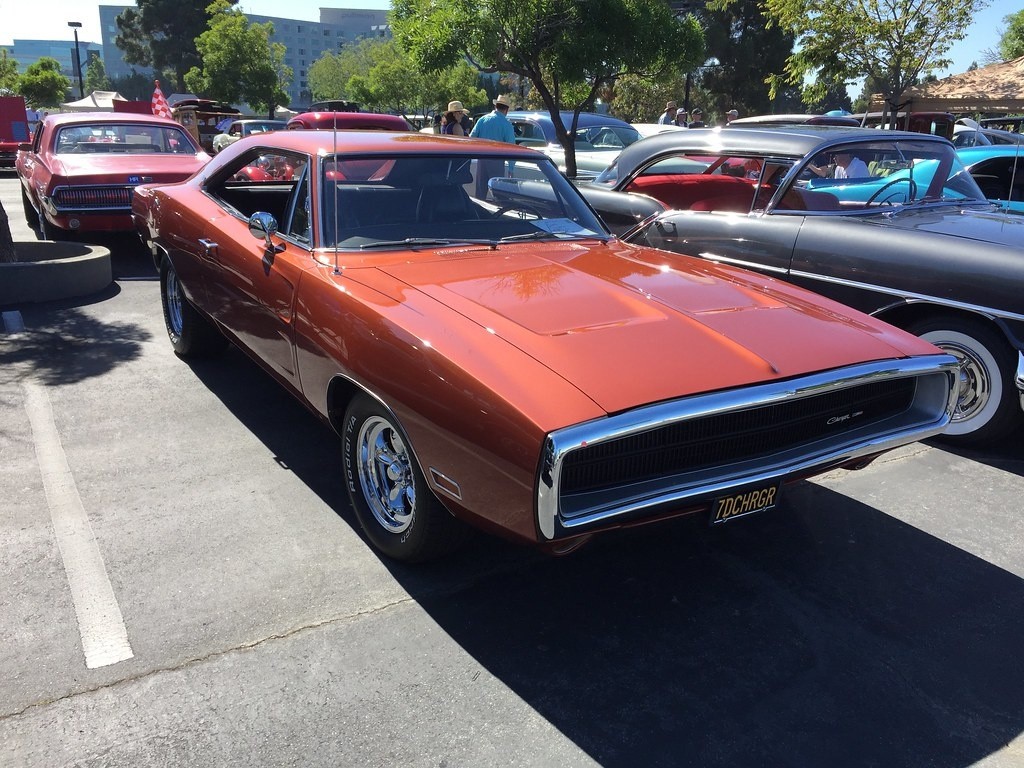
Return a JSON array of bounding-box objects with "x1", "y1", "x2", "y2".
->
[
  {"x1": 859, "y1": 56, "x2": 1024, "y2": 129},
  {"x1": 167, "y1": 94, "x2": 198, "y2": 107},
  {"x1": 268, "y1": 105, "x2": 298, "y2": 117},
  {"x1": 59, "y1": 90, "x2": 129, "y2": 112}
]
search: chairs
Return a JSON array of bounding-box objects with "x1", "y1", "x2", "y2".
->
[
  {"x1": 282, "y1": 179, "x2": 361, "y2": 240},
  {"x1": 416, "y1": 184, "x2": 480, "y2": 228}
]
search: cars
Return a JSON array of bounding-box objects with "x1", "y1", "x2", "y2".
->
[
  {"x1": 489, "y1": 124, "x2": 1024, "y2": 448},
  {"x1": 804, "y1": 144, "x2": 1023, "y2": 214},
  {"x1": 132, "y1": 109, "x2": 962, "y2": 562},
  {"x1": 209, "y1": 119, "x2": 289, "y2": 156},
  {"x1": 234, "y1": 112, "x2": 417, "y2": 182},
  {"x1": 14, "y1": 111, "x2": 213, "y2": 240}
]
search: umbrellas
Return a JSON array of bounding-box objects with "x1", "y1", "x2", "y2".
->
[{"x1": 215, "y1": 117, "x2": 237, "y2": 132}]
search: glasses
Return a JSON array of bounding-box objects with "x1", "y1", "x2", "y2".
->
[{"x1": 455, "y1": 111, "x2": 462, "y2": 114}]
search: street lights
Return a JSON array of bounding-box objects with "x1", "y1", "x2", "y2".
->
[{"x1": 68, "y1": 22, "x2": 84, "y2": 99}]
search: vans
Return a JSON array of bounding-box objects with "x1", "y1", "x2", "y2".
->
[{"x1": 308, "y1": 100, "x2": 359, "y2": 113}]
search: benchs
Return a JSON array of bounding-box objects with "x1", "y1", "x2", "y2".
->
[
  {"x1": 225, "y1": 188, "x2": 416, "y2": 231},
  {"x1": 626, "y1": 175, "x2": 839, "y2": 210}
]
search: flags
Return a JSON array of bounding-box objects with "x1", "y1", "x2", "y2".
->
[{"x1": 151, "y1": 86, "x2": 173, "y2": 120}]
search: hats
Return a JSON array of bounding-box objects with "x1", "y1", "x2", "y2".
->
[
  {"x1": 493, "y1": 95, "x2": 510, "y2": 107},
  {"x1": 664, "y1": 101, "x2": 677, "y2": 112},
  {"x1": 691, "y1": 108, "x2": 703, "y2": 115},
  {"x1": 676, "y1": 108, "x2": 688, "y2": 116},
  {"x1": 443, "y1": 101, "x2": 470, "y2": 115},
  {"x1": 726, "y1": 110, "x2": 738, "y2": 117}
]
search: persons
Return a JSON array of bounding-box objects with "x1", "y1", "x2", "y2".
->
[
  {"x1": 433, "y1": 100, "x2": 473, "y2": 184},
  {"x1": 671, "y1": 108, "x2": 689, "y2": 127},
  {"x1": 27, "y1": 103, "x2": 42, "y2": 132},
  {"x1": 747, "y1": 170, "x2": 760, "y2": 178},
  {"x1": 726, "y1": 110, "x2": 738, "y2": 126},
  {"x1": 689, "y1": 108, "x2": 704, "y2": 128},
  {"x1": 470, "y1": 95, "x2": 517, "y2": 200},
  {"x1": 213, "y1": 133, "x2": 240, "y2": 153},
  {"x1": 806, "y1": 154, "x2": 870, "y2": 180},
  {"x1": 658, "y1": 101, "x2": 678, "y2": 124}
]
